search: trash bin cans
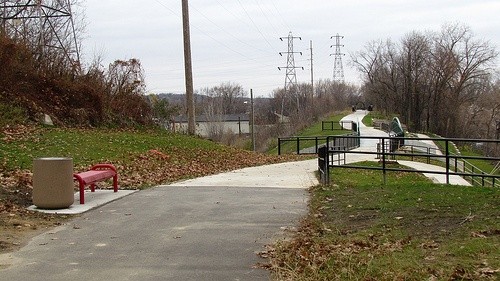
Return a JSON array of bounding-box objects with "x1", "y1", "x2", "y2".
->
[{"x1": 32, "y1": 157, "x2": 76, "y2": 209}]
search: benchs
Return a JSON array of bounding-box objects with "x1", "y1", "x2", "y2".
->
[{"x1": 73, "y1": 164, "x2": 118, "y2": 204}]
